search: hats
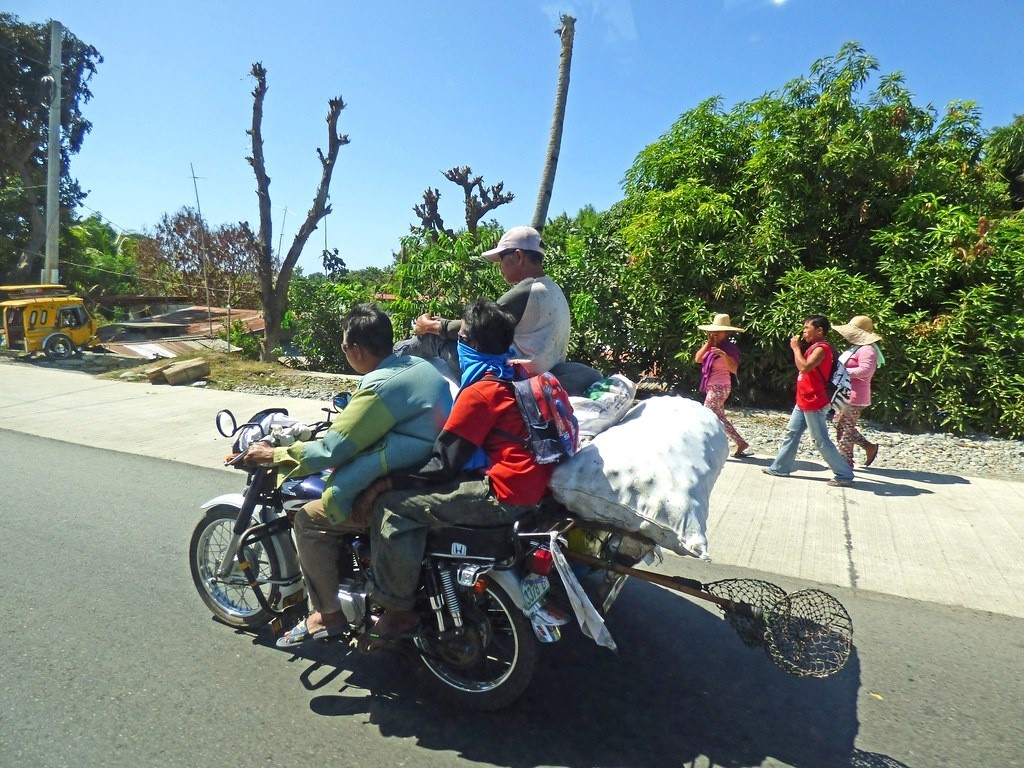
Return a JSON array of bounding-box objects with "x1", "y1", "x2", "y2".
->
[
  {"x1": 696, "y1": 314, "x2": 745, "y2": 333},
  {"x1": 481, "y1": 226, "x2": 546, "y2": 262},
  {"x1": 831, "y1": 315, "x2": 883, "y2": 345}
]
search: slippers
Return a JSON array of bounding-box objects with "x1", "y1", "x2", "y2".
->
[
  {"x1": 275, "y1": 611, "x2": 351, "y2": 648},
  {"x1": 863, "y1": 443, "x2": 879, "y2": 466},
  {"x1": 730, "y1": 449, "x2": 755, "y2": 457},
  {"x1": 356, "y1": 615, "x2": 425, "y2": 655}
]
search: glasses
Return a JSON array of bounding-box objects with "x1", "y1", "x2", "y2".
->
[
  {"x1": 457, "y1": 332, "x2": 476, "y2": 344},
  {"x1": 339, "y1": 342, "x2": 355, "y2": 353},
  {"x1": 500, "y1": 249, "x2": 516, "y2": 260}
]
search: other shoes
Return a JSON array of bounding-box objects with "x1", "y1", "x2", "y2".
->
[
  {"x1": 761, "y1": 467, "x2": 790, "y2": 476},
  {"x1": 828, "y1": 479, "x2": 855, "y2": 487}
]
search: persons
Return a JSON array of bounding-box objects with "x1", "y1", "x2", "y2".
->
[
  {"x1": 242, "y1": 303, "x2": 453, "y2": 646},
  {"x1": 832, "y1": 316, "x2": 878, "y2": 470},
  {"x1": 349, "y1": 297, "x2": 560, "y2": 653},
  {"x1": 392, "y1": 225, "x2": 570, "y2": 384},
  {"x1": 761, "y1": 315, "x2": 855, "y2": 486},
  {"x1": 694, "y1": 314, "x2": 749, "y2": 457}
]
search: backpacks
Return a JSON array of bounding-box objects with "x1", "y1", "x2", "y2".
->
[
  {"x1": 809, "y1": 344, "x2": 853, "y2": 411},
  {"x1": 468, "y1": 367, "x2": 579, "y2": 464}
]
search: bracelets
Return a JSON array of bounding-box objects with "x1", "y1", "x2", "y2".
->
[{"x1": 371, "y1": 483, "x2": 380, "y2": 494}]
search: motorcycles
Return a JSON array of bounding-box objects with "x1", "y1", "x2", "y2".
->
[{"x1": 188, "y1": 389, "x2": 654, "y2": 713}]
